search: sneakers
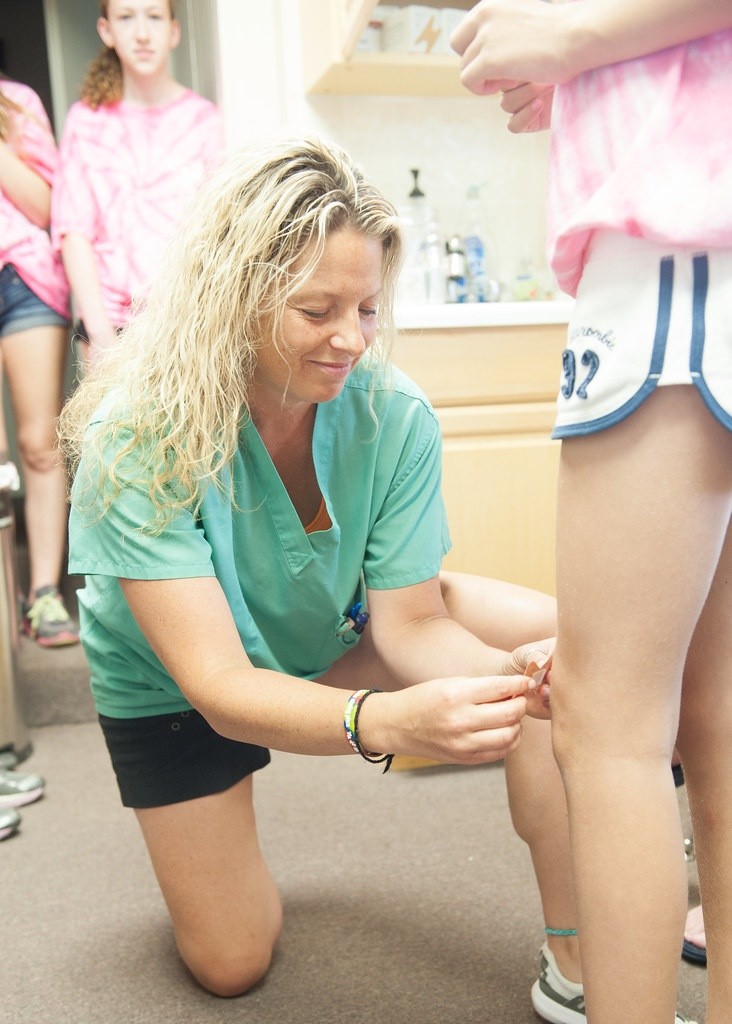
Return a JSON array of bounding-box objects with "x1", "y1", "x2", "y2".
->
[{"x1": 22, "y1": 584, "x2": 79, "y2": 648}]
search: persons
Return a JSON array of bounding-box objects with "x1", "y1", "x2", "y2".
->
[
  {"x1": 0, "y1": 0, "x2": 225, "y2": 840},
  {"x1": 449, "y1": 2, "x2": 732, "y2": 1024},
  {"x1": 55, "y1": 140, "x2": 700, "y2": 1024}
]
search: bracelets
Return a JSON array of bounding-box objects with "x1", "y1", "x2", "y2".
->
[{"x1": 344, "y1": 688, "x2": 396, "y2": 775}]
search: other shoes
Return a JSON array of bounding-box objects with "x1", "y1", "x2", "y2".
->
[
  {"x1": 531, "y1": 941, "x2": 700, "y2": 1024},
  {"x1": 682, "y1": 903, "x2": 707, "y2": 965},
  {"x1": 0, "y1": 767, "x2": 47, "y2": 841}
]
